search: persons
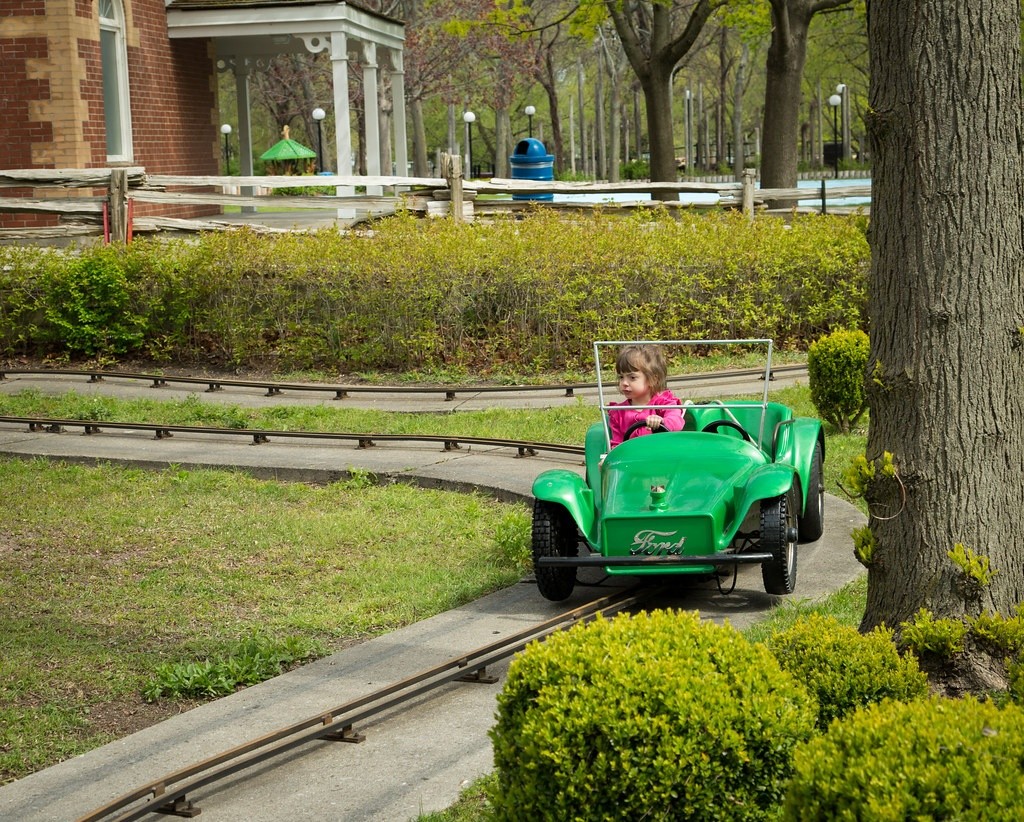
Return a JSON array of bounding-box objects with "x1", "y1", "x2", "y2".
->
[{"x1": 606, "y1": 344, "x2": 685, "y2": 451}]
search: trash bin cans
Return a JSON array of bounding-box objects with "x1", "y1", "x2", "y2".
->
[{"x1": 508, "y1": 139, "x2": 555, "y2": 220}]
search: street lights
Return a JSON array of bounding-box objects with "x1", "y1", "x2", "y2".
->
[
  {"x1": 524, "y1": 106, "x2": 536, "y2": 138},
  {"x1": 829, "y1": 94, "x2": 841, "y2": 179},
  {"x1": 312, "y1": 108, "x2": 326, "y2": 172},
  {"x1": 685, "y1": 90, "x2": 695, "y2": 127},
  {"x1": 463, "y1": 112, "x2": 475, "y2": 179},
  {"x1": 220, "y1": 124, "x2": 232, "y2": 176}
]
